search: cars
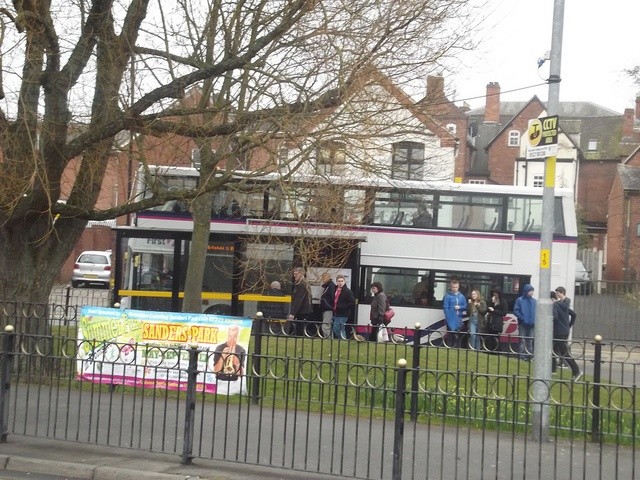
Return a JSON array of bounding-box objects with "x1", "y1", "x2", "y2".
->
[
  {"x1": 574, "y1": 256, "x2": 593, "y2": 296},
  {"x1": 72, "y1": 251, "x2": 112, "y2": 289}
]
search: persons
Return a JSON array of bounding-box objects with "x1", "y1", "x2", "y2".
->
[
  {"x1": 368, "y1": 281, "x2": 391, "y2": 342},
  {"x1": 289, "y1": 267, "x2": 313, "y2": 336},
  {"x1": 420, "y1": 291, "x2": 428, "y2": 304},
  {"x1": 513, "y1": 284, "x2": 537, "y2": 361},
  {"x1": 161, "y1": 186, "x2": 183, "y2": 212},
  {"x1": 414, "y1": 274, "x2": 429, "y2": 302},
  {"x1": 219, "y1": 191, "x2": 241, "y2": 215},
  {"x1": 163, "y1": 267, "x2": 173, "y2": 278},
  {"x1": 555, "y1": 287, "x2": 570, "y2": 369},
  {"x1": 468, "y1": 289, "x2": 488, "y2": 350},
  {"x1": 484, "y1": 286, "x2": 508, "y2": 351},
  {"x1": 550, "y1": 291, "x2": 583, "y2": 381},
  {"x1": 332, "y1": 275, "x2": 355, "y2": 339},
  {"x1": 442, "y1": 280, "x2": 468, "y2": 348},
  {"x1": 412, "y1": 204, "x2": 433, "y2": 228},
  {"x1": 320, "y1": 272, "x2": 336, "y2": 337},
  {"x1": 240, "y1": 194, "x2": 256, "y2": 216},
  {"x1": 213, "y1": 325, "x2": 246, "y2": 395},
  {"x1": 257, "y1": 281, "x2": 289, "y2": 333}
]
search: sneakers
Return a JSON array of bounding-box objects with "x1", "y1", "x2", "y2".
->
[
  {"x1": 574, "y1": 370, "x2": 583, "y2": 382},
  {"x1": 556, "y1": 363, "x2": 569, "y2": 369},
  {"x1": 552, "y1": 371, "x2": 557, "y2": 374}
]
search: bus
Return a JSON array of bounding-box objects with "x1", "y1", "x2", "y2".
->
[{"x1": 121, "y1": 162, "x2": 578, "y2": 351}]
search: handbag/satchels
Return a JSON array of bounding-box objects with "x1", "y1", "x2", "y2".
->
[
  {"x1": 377, "y1": 323, "x2": 389, "y2": 343},
  {"x1": 446, "y1": 308, "x2": 460, "y2": 331},
  {"x1": 384, "y1": 308, "x2": 395, "y2": 322}
]
